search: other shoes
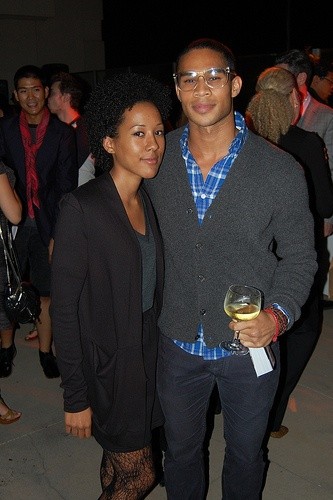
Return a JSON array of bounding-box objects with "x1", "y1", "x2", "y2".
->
[
  {"x1": 0, "y1": 343, "x2": 17, "y2": 378},
  {"x1": 38, "y1": 349, "x2": 60, "y2": 379},
  {"x1": 269, "y1": 425, "x2": 289, "y2": 439},
  {"x1": 24, "y1": 330, "x2": 39, "y2": 341}
]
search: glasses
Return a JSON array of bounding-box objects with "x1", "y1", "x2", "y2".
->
[{"x1": 174, "y1": 67, "x2": 236, "y2": 92}]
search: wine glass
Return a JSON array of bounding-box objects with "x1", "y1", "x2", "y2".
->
[{"x1": 219, "y1": 284, "x2": 261, "y2": 357}]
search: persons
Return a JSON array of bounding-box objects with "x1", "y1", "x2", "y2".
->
[
  {"x1": 0, "y1": 65, "x2": 96, "y2": 379},
  {"x1": 50, "y1": 38, "x2": 332, "y2": 500}
]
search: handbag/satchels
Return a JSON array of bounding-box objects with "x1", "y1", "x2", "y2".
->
[{"x1": 3, "y1": 283, "x2": 43, "y2": 327}]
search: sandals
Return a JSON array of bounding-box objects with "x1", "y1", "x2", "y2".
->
[{"x1": 0, "y1": 408, "x2": 22, "y2": 424}]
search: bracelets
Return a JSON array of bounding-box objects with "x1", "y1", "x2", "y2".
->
[{"x1": 263, "y1": 305, "x2": 287, "y2": 342}]
